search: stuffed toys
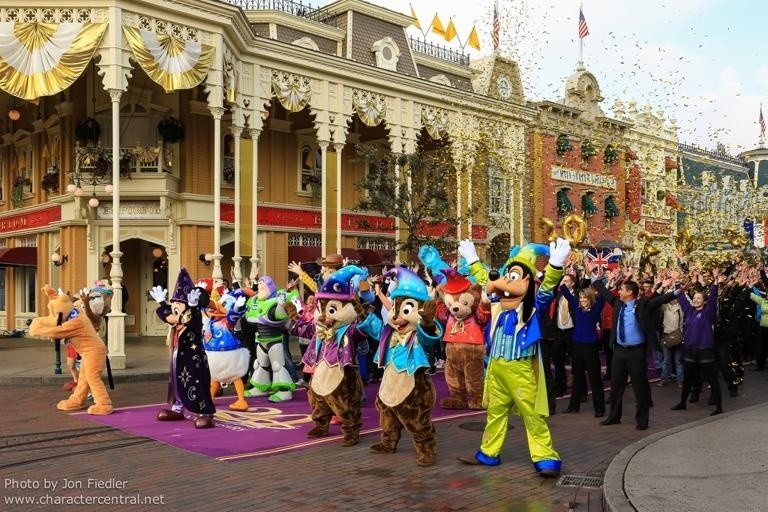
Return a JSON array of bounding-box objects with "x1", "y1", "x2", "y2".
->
[
  {"x1": 149, "y1": 267, "x2": 216, "y2": 428},
  {"x1": 287, "y1": 254, "x2": 348, "y2": 295},
  {"x1": 62, "y1": 281, "x2": 114, "y2": 391},
  {"x1": 457, "y1": 238, "x2": 572, "y2": 477},
  {"x1": 243, "y1": 276, "x2": 299, "y2": 403},
  {"x1": 432, "y1": 269, "x2": 492, "y2": 410},
  {"x1": 354, "y1": 267, "x2": 444, "y2": 467},
  {"x1": 194, "y1": 277, "x2": 248, "y2": 412},
  {"x1": 27, "y1": 285, "x2": 113, "y2": 416},
  {"x1": 305, "y1": 264, "x2": 367, "y2": 447}
]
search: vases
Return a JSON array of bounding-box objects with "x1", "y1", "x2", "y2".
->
[
  {"x1": 224, "y1": 175, "x2": 235, "y2": 183},
  {"x1": 47, "y1": 187, "x2": 59, "y2": 195},
  {"x1": 305, "y1": 185, "x2": 313, "y2": 192}
]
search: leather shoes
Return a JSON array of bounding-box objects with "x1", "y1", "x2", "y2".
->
[
  {"x1": 562, "y1": 407, "x2": 578, "y2": 414},
  {"x1": 671, "y1": 404, "x2": 686, "y2": 411},
  {"x1": 636, "y1": 423, "x2": 648, "y2": 431},
  {"x1": 600, "y1": 418, "x2": 621, "y2": 424},
  {"x1": 710, "y1": 410, "x2": 722, "y2": 416},
  {"x1": 157, "y1": 408, "x2": 185, "y2": 420},
  {"x1": 594, "y1": 412, "x2": 604, "y2": 418},
  {"x1": 195, "y1": 414, "x2": 215, "y2": 429}
]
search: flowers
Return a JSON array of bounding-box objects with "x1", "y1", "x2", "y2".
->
[
  {"x1": 40, "y1": 167, "x2": 60, "y2": 187},
  {"x1": 10, "y1": 177, "x2": 23, "y2": 209},
  {"x1": 302, "y1": 173, "x2": 319, "y2": 184},
  {"x1": 223, "y1": 161, "x2": 236, "y2": 175}
]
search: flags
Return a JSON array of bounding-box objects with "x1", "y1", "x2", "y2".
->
[
  {"x1": 468, "y1": 29, "x2": 480, "y2": 51},
  {"x1": 410, "y1": 7, "x2": 422, "y2": 31},
  {"x1": 759, "y1": 109, "x2": 766, "y2": 130},
  {"x1": 492, "y1": 6, "x2": 500, "y2": 51},
  {"x1": 443, "y1": 21, "x2": 456, "y2": 42},
  {"x1": 430, "y1": 15, "x2": 445, "y2": 36},
  {"x1": 579, "y1": 3, "x2": 590, "y2": 40}
]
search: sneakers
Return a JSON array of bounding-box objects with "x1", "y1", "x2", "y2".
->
[
  {"x1": 455, "y1": 455, "x2": 482, "y2": 465},
  {"x1": 243, "y1": 387, "x2": 268, "y2": 398},
  {"x1": 539, "y1": 469, "x2": 561, "y2": 478},
  {"x1": 269, "y1": 390, "x2": 293, "y2": 403}
]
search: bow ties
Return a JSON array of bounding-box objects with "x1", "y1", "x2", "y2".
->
[
  {"x1": 314, "y1": 324, "x2": 337, "y2": 341},
  {"x1": 389, "y1": 330, "x2": 412, "y2": 349}
]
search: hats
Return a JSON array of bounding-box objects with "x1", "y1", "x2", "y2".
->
[
  {"x1": 505, "y1": 243, "x2": 550, "y2": 276},
  {"x1": 318, "y1": 254, "x2": 344, "y2": 267},
  {"x1": 314, "y1": 266, "x2": 365, "y2": 300},
  {"x1": 440, "y1": 268, "x2": 472, "y2": 293},
  {"x1": 383, "y1": 269, "x2": 434, "y2": 301},
  {"x1": 171, "y1": 268, "x2": 197, "y2": 303},
  {"x1": 227, "y1": 287, "x2": 255, "y2": 299}
]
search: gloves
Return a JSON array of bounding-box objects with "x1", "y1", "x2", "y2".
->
[
  {"x1": 549, "y1": 237, "x2": 572, "y2": 267},
  {"x1": 418, "y1": 301, "x2": 436, "y2": 326},
  {"x1": 187, "y1": 287, "x2": 201, "y2": 307},
  {"x1": 149, "y1": 286, "x2": 167, "y2": 302},
  {"x1": 351, "y1": 299, "x2": 367, "y2": 321},
  {"x1": 458, "y1": 238, "x2": 479, "y2": 264}
]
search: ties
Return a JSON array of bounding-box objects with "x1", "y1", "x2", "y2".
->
[
  {"x1": 561, "y1": 296, "x2": 569, "y2": 326},
  {"x1": 174, "y1": 326, "x2": 182, "y2": 348},
  {"x1": 204, "y1": 319, "x2": 213, "y2": 343},
  {"x1": 619, "y1": 304, "x2": 628, "y2": 343}
]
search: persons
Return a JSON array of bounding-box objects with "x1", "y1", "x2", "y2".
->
[
  {"x1": 586, "y1": 260, "x2": 695, "y2": 432},
  {"x1": 726, "y1": 253, "x2": 766, "y2": 285},
  {"x1": 658, "y1": 282, "x2": 686, "y2": 389},
  {"x1": 546, "y1": 274, "x2": 578, "y2": 399},
  {"x1": 669, "y1": 268, "x2": 724, "y2": 415},
  {"x1": 558, "y1": 283, "x2": 609, "y2": 418},
  {"x1": 747, "y1": 285, "x2": 768, "y2": 352}
]
